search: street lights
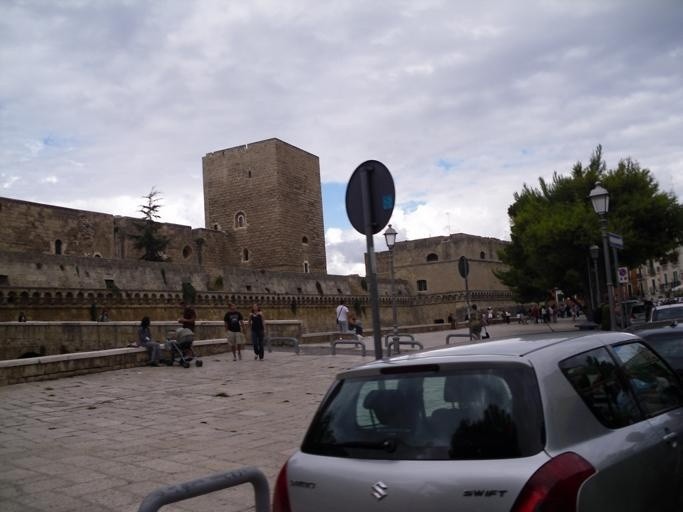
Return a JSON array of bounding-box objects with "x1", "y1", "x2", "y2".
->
[{"x1": 585, "y1": 179, "x2": 619, "y2": 334}]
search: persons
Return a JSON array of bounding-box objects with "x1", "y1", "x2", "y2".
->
[
  {"x1": 447, "y1": 312, "x2": 457, "y2": 330},
  {"x1": 469, "y1": 303, "x2": 483, "y2": 343},
  {"x1": 347, "y1": 311, "x2": 362, "y2": 338},
  {"x1": 246, "y1": 303, "x2": 267, "y2": 360},
  {"x1": 335, "y1": 299, "x2": 347, "y2": 335},
  {"x1": 175, "y1": 301, "x2": 197, "y2": 361},
  {"x1": 164, "y1": 330, "x2": 176, "y2": 357},
  {"x1": 639, "y1": 293, "x2": 683, "y2": 323},
  {"x1": 478, "y1": 301, "x2": 589, "y2": 323},
  {"x1": 137, "y1": 317, "x2": 168, "y2": 366},
  {"x1": 221, "y1": 302, "x2": 247, "y2": 361},
  {"x1": 96, "y1": 308, "x2": 109, "y2": 323}
]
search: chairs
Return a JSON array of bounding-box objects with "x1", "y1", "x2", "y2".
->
[{"x1": 363, "y1": 373, "x2": 488, "y2": 430}]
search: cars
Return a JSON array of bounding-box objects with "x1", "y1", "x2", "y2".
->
[
  {"x1": 267, "y1": 331, "x2": 681, "y2": 511},
  {"x1": 611, "y1": 293, "x2": 681, "y2": 387}
]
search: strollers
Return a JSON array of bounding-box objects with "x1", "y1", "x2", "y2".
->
[{"x1": 164, "y1": 328, "x2": 204, "y2": 369}]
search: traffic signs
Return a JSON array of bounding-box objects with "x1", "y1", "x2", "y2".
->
[
  {"x1": 380, "y1": 223, "x2": 400, "y2": 357},
  {"x1": 607, "y1": 233, "x2": 623, "y2": 249}
]
